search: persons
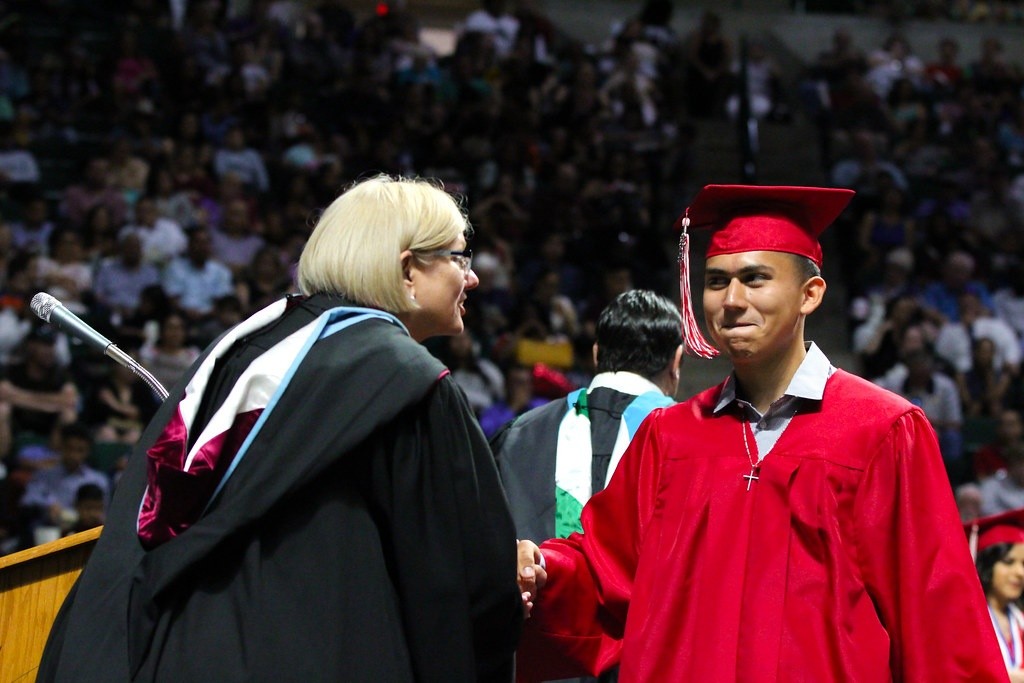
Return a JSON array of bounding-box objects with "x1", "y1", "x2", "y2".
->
[
  {"x1": 489, "y1": 290, "x2": 687, "y2": 545},
  {"x1": 0, "y1": 0, "x2": 1024, "y2": 557},
  {"x1": 964, "y1": 506, "x2": 1024, "y2": 682},
  {"x1": 518, "y1": 184, "x2": 1008, "y2": 683},
  {"x1": 34, "y1": 173, "x2": 527, "y2": 683}
]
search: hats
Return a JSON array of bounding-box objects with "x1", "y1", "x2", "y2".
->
[
  {"x1": 676, "y1": 184, "x2": 857, "y2": 359},
  {"x1": 962, "y1": 509, "x2": 1024, "y2": 564}
]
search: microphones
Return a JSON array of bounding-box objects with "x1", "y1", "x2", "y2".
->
[{"x1": 30, "y1": 293, "x2": 169, "y2": 403}]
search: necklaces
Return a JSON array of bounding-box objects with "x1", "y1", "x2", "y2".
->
[{"x1": 740, "y1": 407, "x2": 799, "y2": 489}]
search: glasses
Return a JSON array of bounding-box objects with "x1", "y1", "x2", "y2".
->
[{"x1": 417, "y1": 247, "x2": 475, "y2": 270}]
